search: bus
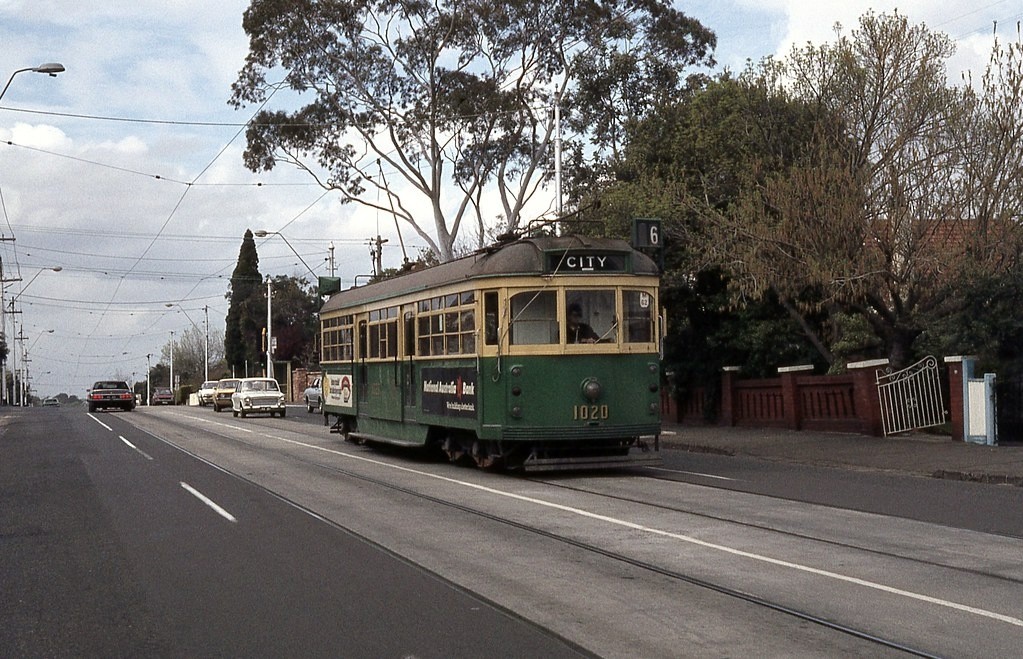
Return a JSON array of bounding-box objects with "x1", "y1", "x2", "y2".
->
[{"x1": 312, "y1": 200, "x2": 663, "y2": 474}]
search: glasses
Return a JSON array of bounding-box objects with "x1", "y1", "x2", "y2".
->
[{"x1": 573, "y1": 312, "x2": 583, "y2": 318}]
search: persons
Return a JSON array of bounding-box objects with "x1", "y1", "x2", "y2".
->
[
  {"x1": 342, "y1": 380, "x2": 350, "y2": 403},
  {"x1": 557, "y1": 303, "x2": 614, "y2": 343},
  {"x1": 322, "y1": 370, "x2": 329, "y2": 401}
]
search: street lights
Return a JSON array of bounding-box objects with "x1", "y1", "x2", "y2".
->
[
  {"x1": 19, "y1": 329, "x2": 55, "y2": 407},
  {"x1": 164, "y1": 303, "x2": 209, "y2": 382},
  {"x1": 11, "y1": 265, "x2": 63, "y2": 406}
]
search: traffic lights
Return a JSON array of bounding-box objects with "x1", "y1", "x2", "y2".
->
[{"x1": 258, "y1": 353, "x2": 267, "y2": 368}]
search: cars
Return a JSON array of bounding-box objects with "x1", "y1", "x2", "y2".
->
[
  {"x1": 85, "y1": 380, "x2": 137, "y2": 412},
  {"x1": 212, "y1": 378, "x2": 241, "y2": 412},
  {"x1": 302, "y1": 376, "x2": 323, "y2": 415},
  {"x1": 42, "y1": 398, "x2": 61, "y2": 407},
  {"x1": 231, "y1": 377, "x2": 286, "y2": 419},
  {"x1": 151, "y1": 387, "x2": 175, "y2": 405},
  {"x1": 197, "y1": 380, "x2": 219, "y2": 407}
]
